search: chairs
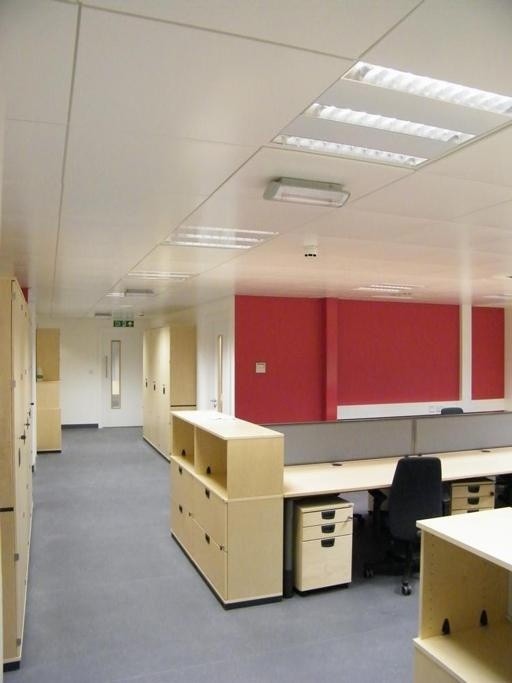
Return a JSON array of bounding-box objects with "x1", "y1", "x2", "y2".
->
[{"x1": 353, "y1": 458, "x2": 446, "y2": 596}]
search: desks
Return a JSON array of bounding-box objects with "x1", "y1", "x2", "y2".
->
[{"x1": 284, "y1": 445, "x2": 512, "y2": 597}]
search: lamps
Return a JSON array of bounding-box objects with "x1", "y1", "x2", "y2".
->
[
  {"x1": 124, "y1": 289, "x2": 154, "y2": 299},
  {"x1": 262, "y1": 178, "x2": 349, "y2": 208},
  {"x1": 95, "y1": 313, "x2": 112, "y2": 319}
]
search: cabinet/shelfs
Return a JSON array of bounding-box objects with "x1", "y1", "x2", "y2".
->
[
  {"x1": 411, "y1": 507, "x2": 512, "y2": 683},
  {"x1": 0, "y1": 276, "x2": 61, "y2": 665},
  {"x1": 450, "y1": 479, "x2": 496, "y2": 515},
  {"x1": 142, "y1": 325, "x2": 195, "y2": 462},
  {"x1": 168, "y1": 410, "x2": 285, "y2": 604},
  {"x1": 293, "y1": 498, "x2": 354, "y2": 591}
]
